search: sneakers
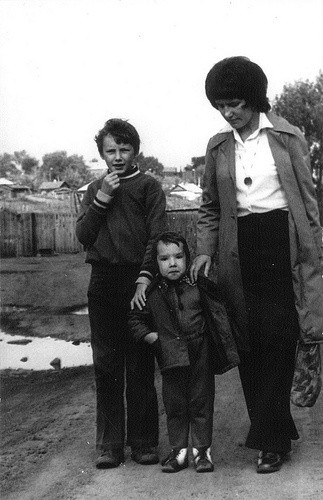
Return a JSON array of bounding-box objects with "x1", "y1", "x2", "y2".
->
[
  {"x1": 192, "y1": 445, "x2": 214, "y2": 472},
  {"x1": 160, "y1": 447, "x2": 188, "y2": 472}
]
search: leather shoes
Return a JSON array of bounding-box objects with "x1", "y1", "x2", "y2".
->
[{"x1": 257, "y1": 451, "x2": 282, "y2": 473}]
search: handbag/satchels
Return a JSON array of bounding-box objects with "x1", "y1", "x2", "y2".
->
[{"x1": 289, "y1": 338, "x2": 321, "y2": 409}]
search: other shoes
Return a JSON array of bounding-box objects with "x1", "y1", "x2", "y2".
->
[
  {"x1": 95, "y1": 447, "x2": 125, "y2": 467},
  {"x1": 130, "y1": 446, "x2": 161, "y2": 464}
]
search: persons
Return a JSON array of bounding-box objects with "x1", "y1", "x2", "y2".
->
[
  {"x1": 125, "y1": 231, "x2": 243, "y2": 471},
  {"x1": 188, "y1": 56, "x2": 323, "y2": 473},
  {"x1": 76, "y1": 118, "x2": 166, "y2": 467}
]
